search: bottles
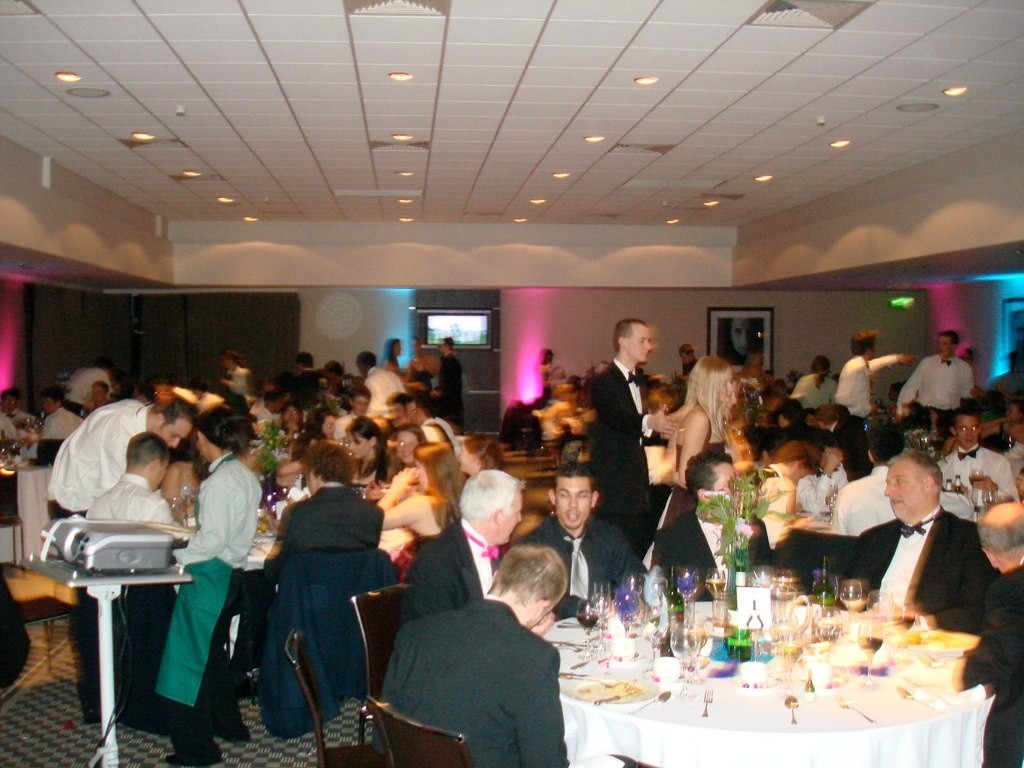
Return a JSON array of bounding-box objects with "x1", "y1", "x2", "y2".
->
[
  {"x1": 664, "y1": 564, "x2": 684, "y2": 652},
  {"x1": 946, "y1": 475, "x2": 961, "y2": 493},
  {"x1": 735, "y1": 428, "x2": 744, "y2": 454},
  {"x1": 814, "y1": 556, "x2": 835, "y2": 617},
  {"x1": 726, "y1": 568, "x2": 751, "y2": 661}
]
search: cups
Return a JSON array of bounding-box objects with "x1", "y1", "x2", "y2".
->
[
  {"x1": 608, "y1": 637, "x2": 636, "y2": 658},
  {"x1": 622, "y1": 572, "x2": 644, "y2": 596},
  {"x1": 867, "y1": 591, "x2": 914, "y2": 632},
  {"x1": 594, "y1": 580, "x2": 610, "y2": 595},
  {"x1": 172, "y1": 494, "x2": 194, "y2": 525},
  {"x1": 677, "y1": 568, "x2": 698, "y2": 598},
  {"x1": 651, "y1": 657, "x2": 684, "y2": 680},
  {"x1": 257, "y1": 509, "x2": 266, "y2": 538},
  {"x1": 771, "y1": 591, "x2": 812, "y2": 634},
  {"x1": 748, "y1": 567, "x2": 795, "y2": 598},
  {"x1": 739, "y1": 661, "x2": 771, "y2": 684},
  {"x1": 969, "y1": 469, "x2": 996, "y2": 506}
]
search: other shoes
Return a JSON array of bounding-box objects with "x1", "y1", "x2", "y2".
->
[
  {"x1": 166, "y1": 740, "x2": 222, "y2": 765},
  {"x1": 211, "y1": 720, "x2": 251, "y2": 742}
]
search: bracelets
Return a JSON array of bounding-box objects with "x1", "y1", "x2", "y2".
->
[{"x1": 822, "y1": 471, "x2": 831, "y2": 478}]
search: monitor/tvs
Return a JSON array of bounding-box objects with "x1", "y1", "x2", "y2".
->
[{"x1": 415, "y1": 309, "x2": 501, "y2": 351}]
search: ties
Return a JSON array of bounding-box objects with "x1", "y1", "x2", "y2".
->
[
  {"x1": 227, "y1": 366, "x2": 236, "y2": 380},
  {"x1": 563, "y1": 534, "x2": 591, "y2": 600}
]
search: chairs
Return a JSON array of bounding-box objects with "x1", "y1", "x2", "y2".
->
[{"x1": 0, "y1": 400, "x2": 1024, "y2": 768}]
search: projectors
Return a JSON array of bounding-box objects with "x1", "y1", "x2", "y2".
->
[{"x1": 41, "y1": 518, "x2": 175, "y2": 569}]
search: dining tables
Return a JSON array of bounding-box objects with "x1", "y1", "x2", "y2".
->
[
  {"x1": 543, "y1": 598, "x2": 997, "y2": 767},
  {"x1": 0, "y1": 464, "x2": 52, "y2": 564},
  {"x1": 173, "y1": 509, "x2": 412, "y2": 680}
]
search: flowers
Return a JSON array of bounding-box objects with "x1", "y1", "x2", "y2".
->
[
  {"x1": 695, "y1": 467, "x2": 795, "y2": 569},
  {"x1": 249, "y1": 423, "x2": 289, "y2": 478}
]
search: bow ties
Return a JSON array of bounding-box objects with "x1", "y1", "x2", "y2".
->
[
  {"x1": 940, "y1": 358, "x2": 953, "y2": 366},
  {"x1": 898, "y1": 516, "x2": 936, "y2": 538},
  {"x1": 463, "y1": 530, "x2": 501, "y2": 564},
  {"x1": 626, "y1": 372, "x2": 644, "y2": 388},
  {"x1": 956, "y1": 445, "x2": 981, "y2": 461}
]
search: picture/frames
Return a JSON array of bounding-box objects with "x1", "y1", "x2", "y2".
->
[
  {"x1": 1001, "y1": 296, "x2": 1024, "y2": 364},
  {"x1": 706, "y1": 307, "x2": 774, "y2": 376}
]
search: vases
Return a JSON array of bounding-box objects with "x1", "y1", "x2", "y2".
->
[
  {"x1": 265, "y1": 472, "x2": 282, "y2": 512},
  {"x1": 726, "y1": 548, "x2": 749, "y2": 601}
]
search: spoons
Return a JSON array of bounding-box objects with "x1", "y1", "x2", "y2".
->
[
  {"x1": 784, "y1": 697, "x2": 798, "y2": 725},
  {"x1": 628, "y1": 691, "x2": 671, "y2": 715}
]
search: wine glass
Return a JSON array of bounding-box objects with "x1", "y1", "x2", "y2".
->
[
  {"x1": 840, "y1": 581, "x2": 861, "y2": 620},
  {"x1": 613, "y1": 590, "x2": 639, "y2": 638},
  {"x1": 590, "y1": 594, "x2": 613, "y2": 651},
  {"x1": 814, "y1": 607, "x2": 843, "y2": 665},
  {"x1": 857, "y1": 620, "x2": 883, "y2": 692},
  {"x1": 776, "y1": 633, "x2": 804, "y2": 697},
  {"x1": 576, "y1": 598, "x2": 600, "y2": 659},
  {"x1": 670, "y1": 622, "x2": 701, "y2": 699},
  {"x1": 705, "y1": 567, "x2": 726, "y2": 621}
]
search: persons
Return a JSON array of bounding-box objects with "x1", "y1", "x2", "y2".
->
[
  {"x1": 588, "y1": 318, "x2": 677, "y2": 555},
  {"x1": 835, "y1": 330, "x2": 917, "y2": 421},
  {"x1": 518, "y1": 460, "x2": 650, "y2": 622},
  {"x1": 639, "y1": 354, "x2": 739, "y2": 570},
  {"x1": 825, "y1": 447, "x2": 998, "y2": 636},
  {"x1": 928, "y1": 408, "x2": 1020, "y2": 514},
  {"x1": 0, "y1": 324, "x2": 1024, "y2": 768},
  {"x1": 430, "y1": 336, "x2": 464, "y2": 433},
  {"x1": 789, "y1": 355, "x2": 837, "y2": 410},
  {"x1": 652, "y1": 447, "x2": 816, "y2": 599},
  {"x1": 893, "y1": 331, "x2": 981, "y2": 420},
  {"x1": 85, "y1": 433, "x2": 185, "y2": 594},
  {"x1": 400, "y1": 468, "x2": 554, "y2": 638},
  {"x1": 277, "y1": 441, "x2": 385, "y2": 567}
]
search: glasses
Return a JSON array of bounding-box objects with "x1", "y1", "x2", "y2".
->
[
  {"x1": 4, "y1": 398, "x2": 17, "y2": 406},
  {"x1": 956, "y1": 424, "x2": 979, "y2": 432},
  {"x1": 398, "y1": 441, "x2": 415, "y2": 447},
  {"x1": 1008, "y1": 408, "x2": 1020, "y2": 414},
  {"x1": 323, "y1": 423, "x2": 334, "y2": 429}
]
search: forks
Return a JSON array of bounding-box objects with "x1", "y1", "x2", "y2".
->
[
  {"x1": 838, "y1": 699, "x2": 875, "y2": 724},
  {"x1": 702, "y1": 689, "x2": 713, "y2": 717}
]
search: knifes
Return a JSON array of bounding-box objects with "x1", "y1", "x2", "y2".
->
[
  {"x1": 594, "y1": 692, "x2": 636, "y2": 705},
  {"x1": 897, "y1": 687, "x2": 943, "y2": 714}
]
